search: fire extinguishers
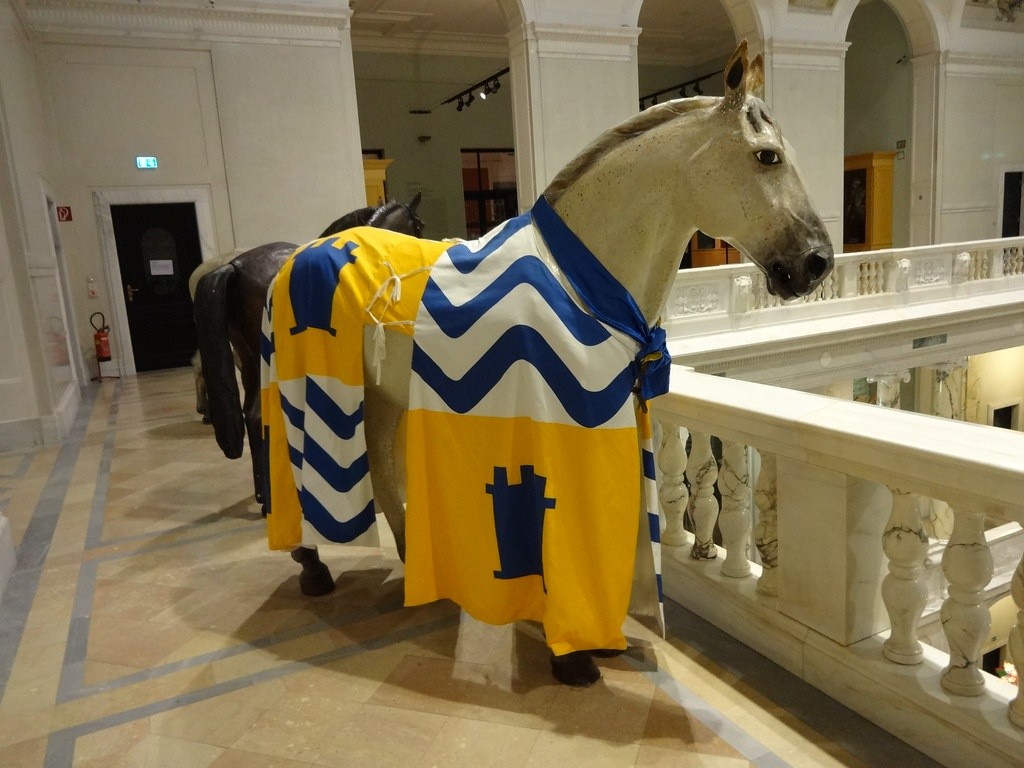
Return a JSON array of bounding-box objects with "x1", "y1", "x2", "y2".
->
[{"x1": 90, "y1": 312, "x2": 112, "y2": 380}]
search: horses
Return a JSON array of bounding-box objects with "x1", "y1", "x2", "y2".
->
[{"x1": 181, "y1": 37, "x2": 834, "y2": 694}]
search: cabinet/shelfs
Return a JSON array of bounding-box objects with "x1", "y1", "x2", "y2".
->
[
  {"x1": 843, "y1": 151, "x2": 898, "y2": 253},
  {"x1": 691, "y1": 230, "x2": 741, "y2": 268},
  {"x1": 362, "y1": 159, "x2": 395, "y2": 207}
]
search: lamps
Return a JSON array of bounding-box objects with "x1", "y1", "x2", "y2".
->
[
  {"x1": 639, "y1": 69, "x2": 725, "y2": 111},
  {"x1": 441, "y1": 66, "x2": 510, "y2": 112}
]
